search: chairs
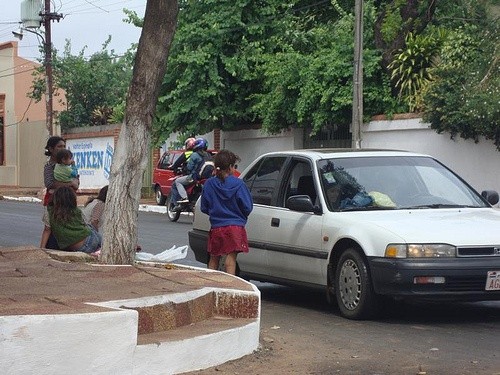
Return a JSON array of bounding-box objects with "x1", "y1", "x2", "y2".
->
[{"x1": 297, "y1": 176, "x2": 316, "y2": 204}]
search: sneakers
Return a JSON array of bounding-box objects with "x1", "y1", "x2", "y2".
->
[
  {"x1": 172, "y1": 204, "x2": 181, "y2": 211},
  {"x1": 176, "y1": 198, "x2": 189, "y2": 203}
]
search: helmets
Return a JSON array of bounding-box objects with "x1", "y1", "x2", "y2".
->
[
  {"x1": 185, "y1": 137, "x2": 196, "y2": 150},
  {"x1": 192, "y1": 137, "x2": 208, "y2": 153}
]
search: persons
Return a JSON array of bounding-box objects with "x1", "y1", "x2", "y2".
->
[
  {"x1": 83, "y1": 185, "x2": 142, "y2": 251},
  {"x1": 167, "y1": 138, "x2": 196, "y2": 211},
  {"x1": 43, "y1": 135, "x2": 80, "y2": 249},
  {"x1": 175, "y1": 138, "x2": 211, "y2": 203},
  {"x1": 40, "y1": 186, "x2": 102, "y2": 254},
  {"x1": 43, "y1": 149, "x2": 79, "y2": 205},
  {"x1": 200, "y1": 149, "x2": 253, "y2": 276},
  {"x1": 315, "y1": 178, "x2": 342, "y2": 211}
]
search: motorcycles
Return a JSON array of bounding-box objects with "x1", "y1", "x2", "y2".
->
[{"x1": 167, "y1": 164, "x2": 206, "y2": 222}]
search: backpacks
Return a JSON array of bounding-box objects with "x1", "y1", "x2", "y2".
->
[{"x1": 191, "y1": 151, "x2": 215, "y2": 183}]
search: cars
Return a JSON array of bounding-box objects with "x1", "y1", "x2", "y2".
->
[
  {"x1": 151, "y1": 150, "x2": 241, "y2": 205},
  {"x1": 189, "y1": 148, "x2": 500, "y2": 320}
]
